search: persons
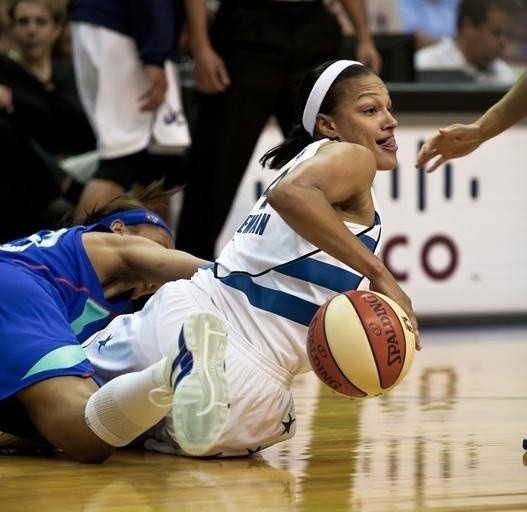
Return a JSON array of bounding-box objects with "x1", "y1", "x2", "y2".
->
[
  {"x1": 414, "y1": 1, "x2": 518, "y2": 85},
  {"x1": 73, "y1": 57, "x2": 423, "y2": 461},
  {"x1": 0, "y1": 181, "x2": 217, "y2": 461},
  {"x1": 416, "y1": 71, "x2": 526, "y2": 173},
  {"x1": 1, "y1": 2, "x2": 380, "y2": 259}
]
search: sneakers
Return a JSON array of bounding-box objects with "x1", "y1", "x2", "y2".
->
[{"x1": 163, "y1": 311, "x2": 229, "y2": 456}]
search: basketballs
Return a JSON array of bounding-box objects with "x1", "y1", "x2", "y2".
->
[{"x1": 308, "y1": 289, "x2": 416, "y2": 399}]
